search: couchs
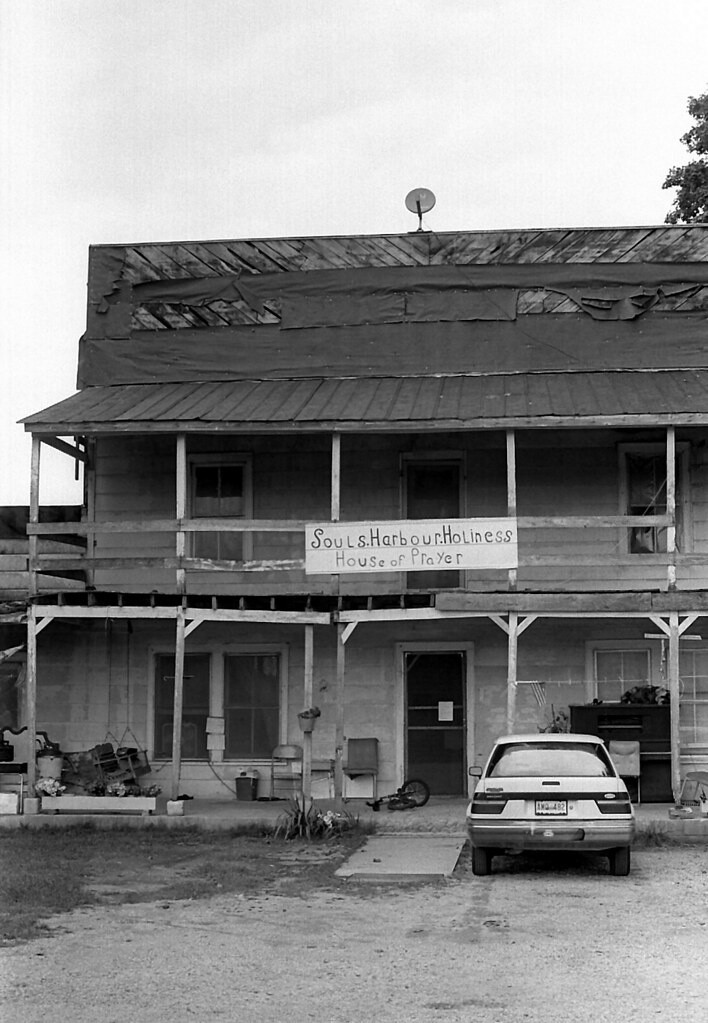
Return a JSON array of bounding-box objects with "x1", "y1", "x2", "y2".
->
[{"x1": 0, "y1": 726, "x2": 51, "y2": 795}]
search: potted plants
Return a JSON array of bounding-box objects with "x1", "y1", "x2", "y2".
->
[{"x1": 297, "y1": 707, "x2": 321, "y2": 732}]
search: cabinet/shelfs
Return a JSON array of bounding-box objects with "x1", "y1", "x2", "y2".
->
[{"x1": 569, "y1": 704, "x2": 674, "y2": 803}]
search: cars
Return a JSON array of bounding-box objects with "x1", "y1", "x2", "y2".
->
[{"x1": 465, "y1": 732, "x2": 635, "y2": 878}]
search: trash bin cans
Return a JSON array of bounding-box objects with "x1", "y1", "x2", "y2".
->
[
  {"x1": 36, "y1": 749, "x2": 63, "y2": 784},
  {"x1": 234, "y1": 776, "x2": 258, "y2": 802}
]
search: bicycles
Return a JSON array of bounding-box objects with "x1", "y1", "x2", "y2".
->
[{"x1": 366, "y1": 779, "x2": 432, "y2": 812}]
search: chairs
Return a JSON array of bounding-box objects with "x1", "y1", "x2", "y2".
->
[
  {"x1": 608, "y1": 740, "x2": 642, "y2": 804},
  {"x1": 680, "y1": 772, "x2": 708, "y2": 809},
  {"x1": 343, "y1": 737, "x2": 379, "y2": 803},
  {"x1": 269, "y1": 745, "x2": 303, "y2": 801}
]
